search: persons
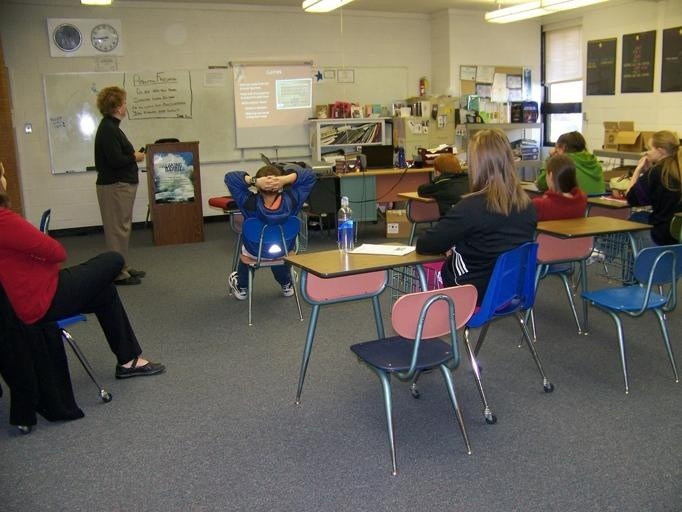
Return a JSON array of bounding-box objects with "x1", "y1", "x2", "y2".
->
[
  {"x1": 416, "y1": 122, "x2": 538, "y2": 314},
  {"x1": 221, "y1": 161, "x2": 318, "y2": 300},
  {"x1": 532, "y1": 130, "x2": 608, "y2": 197},
  {"x1": 417, "y1": 150, "x2": 473, "y2": 219},
  {"x1": 91, "y1": 86, "x2": 146, "y2": 284},
  {"x1": 627, "y1": 131, "x2": 682, "y2": 247},
  {"x1": 531, "y1": 152, "x2": 589, "y2": 221},
  {"x1": 1, "y1": 160, "x2": 165, "y2": 380}
]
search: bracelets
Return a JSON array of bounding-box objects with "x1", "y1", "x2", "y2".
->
[{"x1": 636, "y1": 167, "x2": 642, "y2": 173}]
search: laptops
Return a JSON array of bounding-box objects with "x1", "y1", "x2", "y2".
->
[{"x1": 362, "y1": 144, "x2": 394, "y2": 169}]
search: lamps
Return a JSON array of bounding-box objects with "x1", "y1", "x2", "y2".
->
[
  {"x1": 483, "y1": 0, "x2": 617, "y2": 26},
  {"x1": 296, "y1": 1, "x2": 354, "y2": 14}
]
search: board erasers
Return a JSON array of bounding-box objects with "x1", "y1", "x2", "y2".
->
[{"x1": 86, "y1": 167, "x2": 96, "y2": 171}]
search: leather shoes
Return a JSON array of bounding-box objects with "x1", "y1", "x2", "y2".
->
[
  {"x1": 115, "y1": 359, "x2": 165, "y2": 380},
  {"x1": 112, "y1": 277, "x2": 142, "y2": 286},
  {"x1": 127, "y1": 268, "x2": 146, "y2": 278}
]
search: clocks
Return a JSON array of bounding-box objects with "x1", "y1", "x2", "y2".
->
[{"x1": 90, "y1": 24, "x2": 120, "y2": 53}]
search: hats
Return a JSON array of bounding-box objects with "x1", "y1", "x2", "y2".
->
[{"x1": 425, "y1": 153, "x2": 461, "y2": 173}]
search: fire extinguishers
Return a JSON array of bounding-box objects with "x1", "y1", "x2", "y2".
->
[{"x1": 419, "y1": 76, "x2": 427, "y2": 96}]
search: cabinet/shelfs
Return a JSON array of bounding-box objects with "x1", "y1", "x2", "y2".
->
[
  {"x1": 458, "y1": 122, "x2": 541, "y2": 184},
  {"x1": 312, "y1": 120, "x2": 385, "y2": 159},
  {"x1": 396, "y1": 99, "x2": 457, "y2": 157}
]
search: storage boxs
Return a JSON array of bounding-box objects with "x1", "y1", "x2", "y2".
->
[
  {"x1": 510, "y1": 101, "x2": 539, "y2": 123},
  {"x1": 603, "y1": 118, "x2": 682, "y2": 181},
  {"x1": 515, "y1": 143, "x2": 539, "y2": 160}
]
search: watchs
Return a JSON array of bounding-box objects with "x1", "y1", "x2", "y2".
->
[{"x1": 250, "y1": 176, "x2": 257, "y2": 187}]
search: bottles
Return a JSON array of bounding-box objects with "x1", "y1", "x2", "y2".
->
[
  {"x1": 419, "y1": 75, "x2": 430, "y2": 96},
  {"x1": 336, "y1": 195, "x2": 355, "y2": 250}
]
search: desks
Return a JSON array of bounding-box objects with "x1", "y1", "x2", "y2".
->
[{"x1": 340, "y1": 167, "x2": 436, "y2": 244}]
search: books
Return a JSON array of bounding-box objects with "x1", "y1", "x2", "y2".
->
[
  {"x1": 320, "y1": 122, "x2": 382, "y2": 144},
  {"x1": 415, "y1": 101, "x2": 419, "y2": 116},
  {"x1": 419, "y1": 102, "x2": 430, "y2": 118},
  {"x1": 510, "y1": 138, "x2": 539, "y2": 161}
]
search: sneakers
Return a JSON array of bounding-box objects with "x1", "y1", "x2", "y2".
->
[
  {"x1": 586, "y1": 246, "x2": 604, "y2": 265},
  {"x1": 229, "y1": 271, "x2": 248, "y2": 301},
  {"x1": 282, "y1": 270, "x2": 298, "y2": 297}
]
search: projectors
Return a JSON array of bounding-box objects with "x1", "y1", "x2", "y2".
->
[{"x1": 310, "y1": 162, "x2": 336, "y2": 175}]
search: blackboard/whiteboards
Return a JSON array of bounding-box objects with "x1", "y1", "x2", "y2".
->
[{"x1": 42, "y1": 64, "x2": 409, "y2": 176}]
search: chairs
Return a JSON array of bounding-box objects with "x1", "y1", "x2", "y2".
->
[{"x1": 0, "y1": 208, "x2": 116, "y2": 431}]
